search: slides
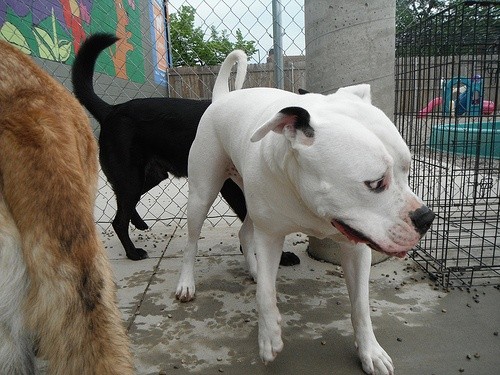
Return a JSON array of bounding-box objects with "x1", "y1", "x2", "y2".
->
[{"x1": 417, "y1": 96, "x2": 442, "y2": 116}]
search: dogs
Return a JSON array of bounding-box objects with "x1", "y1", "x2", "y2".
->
[
  {"x1": 0, "y1": 40, "x2": 133, "y2": 374},
  {"x1": 175, "y1": 50, "x2": 435, "y2": 375},
  {"x1": 71, "y1": 32, "x2": 311, "y2": 267}
]
